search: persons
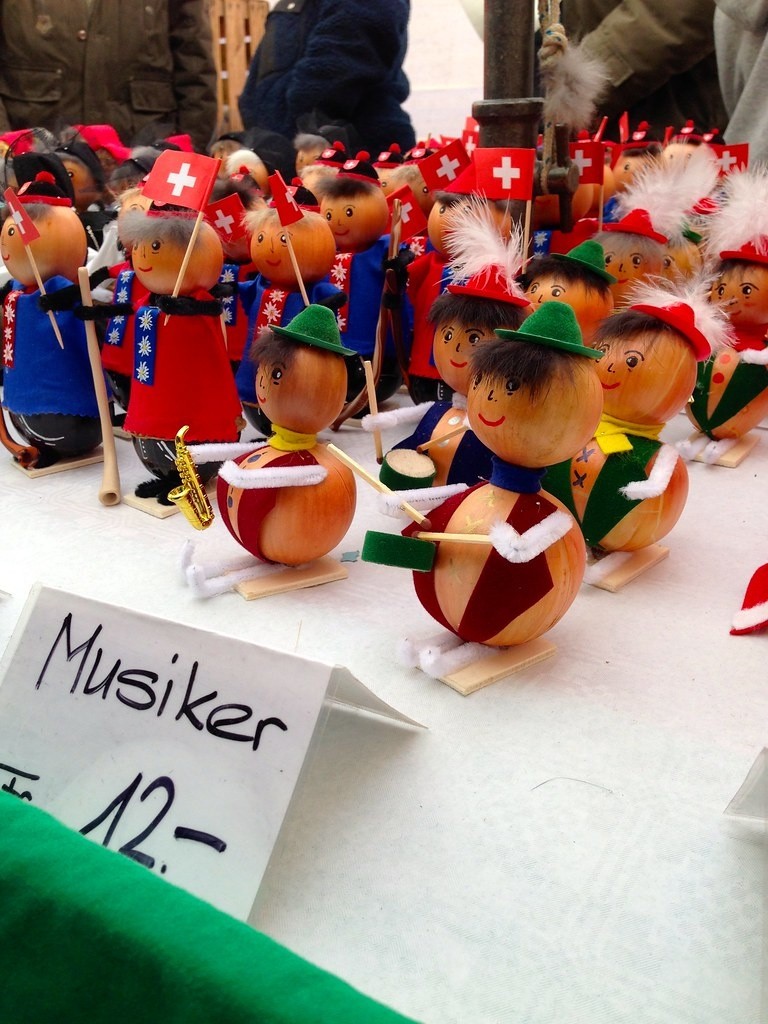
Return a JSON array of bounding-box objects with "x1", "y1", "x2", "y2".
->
[
  {"x1": 532, "y1": 14, "x2": 555, "y2": 132},
  {"x1": 0, "y1": 0, "x2": 219, "y2": 163},
  {"x1": 554, "y1": 0, "x2": 726, "y2": 147},
  {"x1": 559, "y1": 0, "x2": 768, "y2": 206},
  {"x1": 237, "y1": 0, "x2": 416, "y2": 180}
]
